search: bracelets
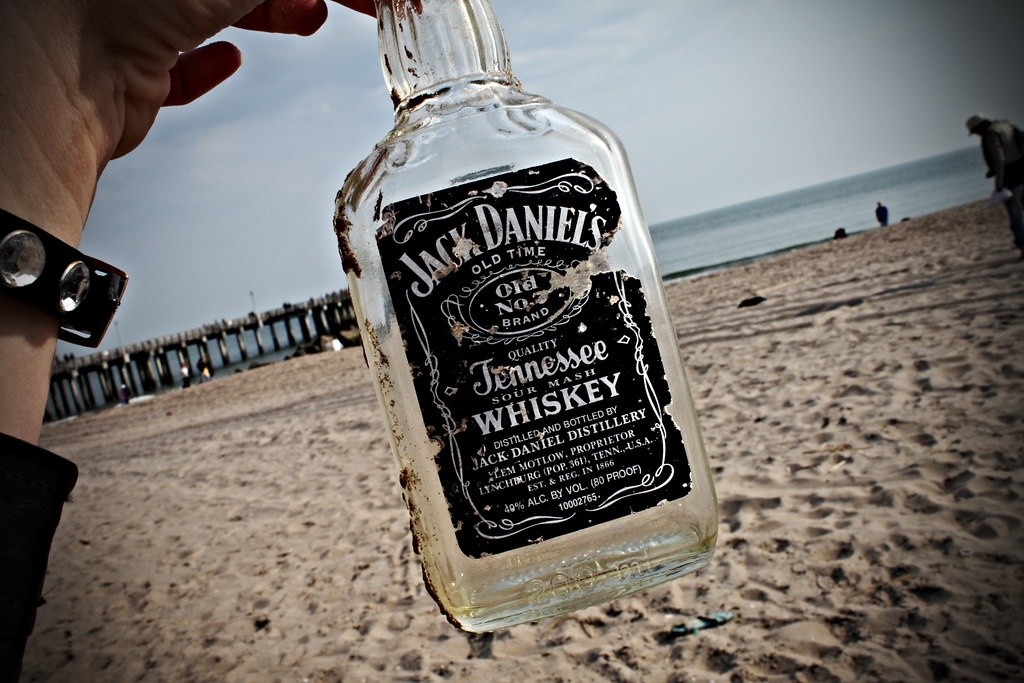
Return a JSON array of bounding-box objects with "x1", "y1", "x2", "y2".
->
[{"x1": 0, "y1": 209, "x2": 129, "y2": 348}]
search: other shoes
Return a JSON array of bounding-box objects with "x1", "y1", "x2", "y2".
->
[{"x1": 989, "y1": 187, "x2": 1012, "y2": 207}]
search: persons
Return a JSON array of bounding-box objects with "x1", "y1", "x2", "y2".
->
[
  {"x1": 965, "y1": 114, "x2": 1024, "y2": 260},
  {"x1": 0, "y1": 0, "x2": 378, "y2": 683},
  {"x1": 876, "y1": 202, "x2": 888, "y2": 226}
]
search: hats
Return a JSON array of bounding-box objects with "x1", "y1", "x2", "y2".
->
[{"x1": 966, "y1": 115, "x2": 985, "y2": 135}]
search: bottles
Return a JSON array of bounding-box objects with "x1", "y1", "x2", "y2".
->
[{"x1": 333, "y1": 0, "x2": 717, "y2": 634}]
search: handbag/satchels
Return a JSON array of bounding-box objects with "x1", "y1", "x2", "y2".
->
[{"x1": 1011, "y1": 125, "x2": 1024, "y2": 155}]
